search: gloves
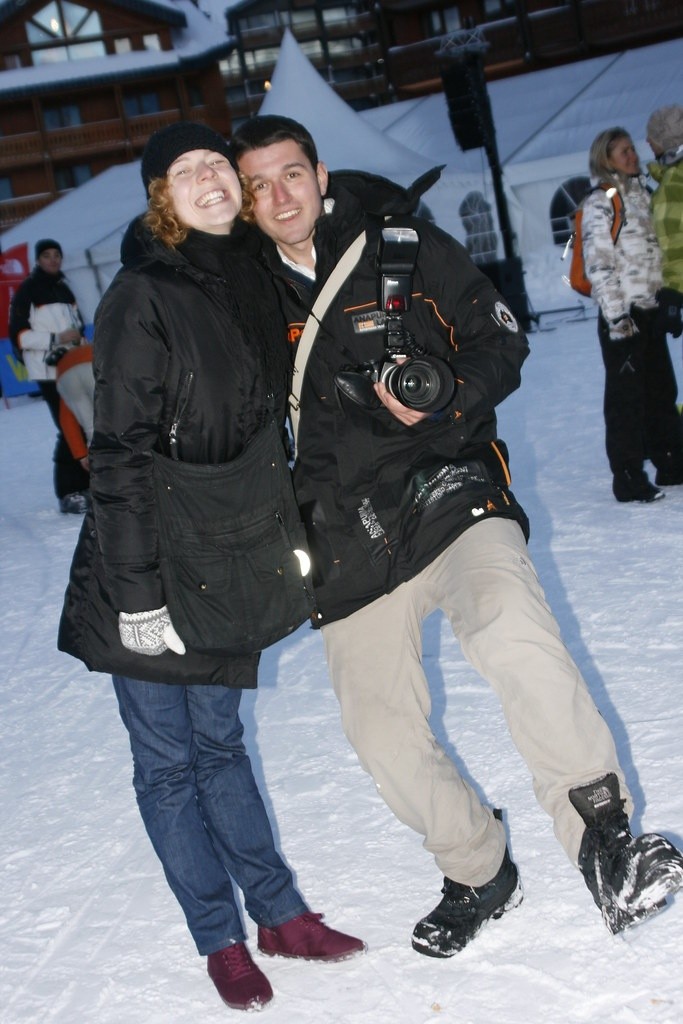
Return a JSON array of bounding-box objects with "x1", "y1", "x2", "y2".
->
[
  {"x1": 608, "y1": 315, "x2": 640, "y2": 341},
  {"x1": 118, "y1": 606, "x2": 187, "y2": 655},
  {"x1": 654, "y1": 286, "x2": 683, "y2": 339}
]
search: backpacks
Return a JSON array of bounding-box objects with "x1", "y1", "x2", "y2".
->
[{"x1": 567, "y1": 184, "x2": 629, "y2": 297}]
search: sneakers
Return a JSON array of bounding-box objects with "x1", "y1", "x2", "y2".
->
[
  {"x1": 568, "y1": 773, "x2": 683, "y2": 935},
  {"x1": 208, "y1": 937, "x2": 274, "y2": 1010},
  {"x1": 257, "y1": 909, "x2": 367, "y2": 964},
  {"x1": 409, "y1": 809, "x2": 524, "y2": 958}
]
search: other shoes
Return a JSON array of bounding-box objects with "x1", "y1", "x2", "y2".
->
[
  {"x1": 612, "y1": 473, "x2": 663, "y2": 501},
  {"x1": 57, "y1": 492, "x2": 90, "y2": 513},
  {"x1": 650, "y1": 455, "x2": 683, "y2": 486}
]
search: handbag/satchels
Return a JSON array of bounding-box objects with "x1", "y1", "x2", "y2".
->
[{"x1": 152, "y1": 418, "x2": 319, "y2": 651}]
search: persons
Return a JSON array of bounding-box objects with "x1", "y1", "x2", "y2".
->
[
  {"x1": 225, "y1": 117, "x2": 683, "y2": 959},
  {"x1": 645, "y1": 106, "x2": 683, "y2": 435},
  {"x1": 58, "y1": 119, "x2": 365, "y2": 1012},
  {"x1": 8, "y1": 239, "x2": 99, "y2": 513},
  {"x1": 568, "y1": 126, "x2": 683, "y2": 505}
]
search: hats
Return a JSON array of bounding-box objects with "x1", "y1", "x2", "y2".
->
[
  {"x1": 140, "y1": 121, "x2": 239, "y2": 199},
  {"x1": 34, "y1": 239, "x2": 63, "y2": 260}
]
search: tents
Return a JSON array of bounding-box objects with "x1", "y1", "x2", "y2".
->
[
  {"x1": 0, "y1": 159, "x2": 149, "y2": 326},
  {"x1": 257, "y1": 27, "x2": 540, "y2": 334}
]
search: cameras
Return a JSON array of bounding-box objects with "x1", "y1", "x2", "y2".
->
[
  {"x1": 372, "y1": 228, "x2": 455, "y2": 412},
  {"x1": 45, "y1": 346, "x2": 69, "y2": 365}
]
